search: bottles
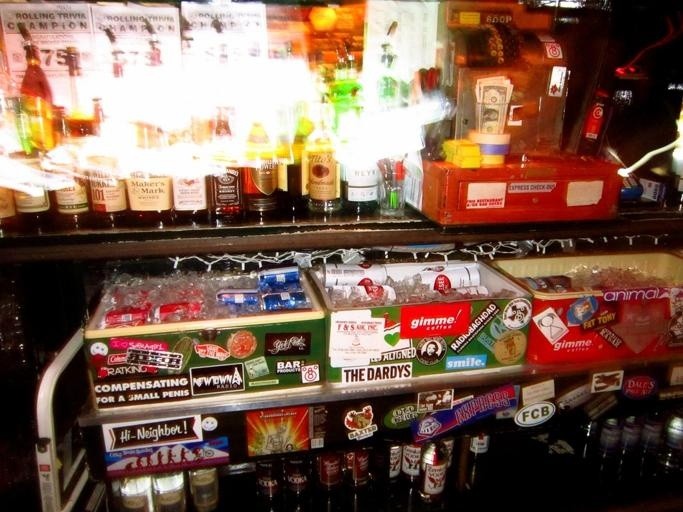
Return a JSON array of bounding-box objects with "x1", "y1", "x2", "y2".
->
[
  {"x1": 0, "y1": 35, "x2": 415, "y2": 229},
  {"x1": 119, "y1": 413, "x2": 683, "y2": 512}
]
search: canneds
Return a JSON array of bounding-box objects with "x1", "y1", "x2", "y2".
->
[{"x1": 103, "y1": 265, "x2": 606, "y2": 326}]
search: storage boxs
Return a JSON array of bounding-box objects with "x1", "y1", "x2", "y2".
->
[
  {"x1": 490, "y1": 252, "x2": 681, "y2": 372},
  {"x1": 84, "y1": 263, "x2": 328, "y2": 393},
  {"x1": 308, "y1": 257, "x2": 533, "y2": 384}
]
